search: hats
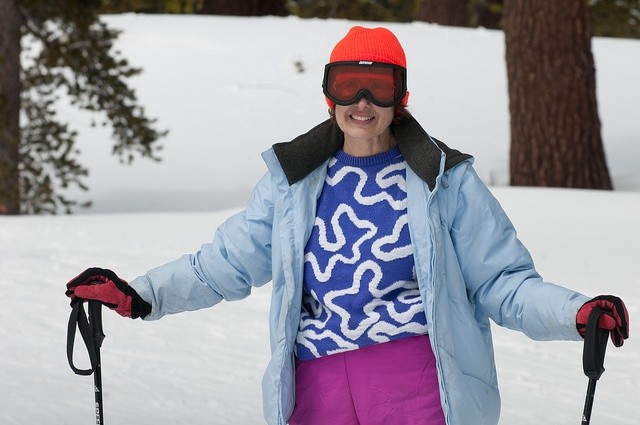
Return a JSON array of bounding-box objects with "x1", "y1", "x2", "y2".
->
[{"x1": 326, "y1": 25, "x2": 411, "y2": 109}]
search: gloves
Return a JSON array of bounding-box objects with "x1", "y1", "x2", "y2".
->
[
  {"x1": 65, "y1": 266, "x2": 140, "y2": 319},
  {"x1": 575, "y1": 295, "x2": 632, "y2": 347}
]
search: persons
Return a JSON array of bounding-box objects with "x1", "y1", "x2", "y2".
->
[{"x1": 65, "y1": 27, "x2": 628, "y2": 425}]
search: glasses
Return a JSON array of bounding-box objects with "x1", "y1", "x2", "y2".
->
[{"x1": 323, "y1": 57, "x2": 408, "y2": 106}]
